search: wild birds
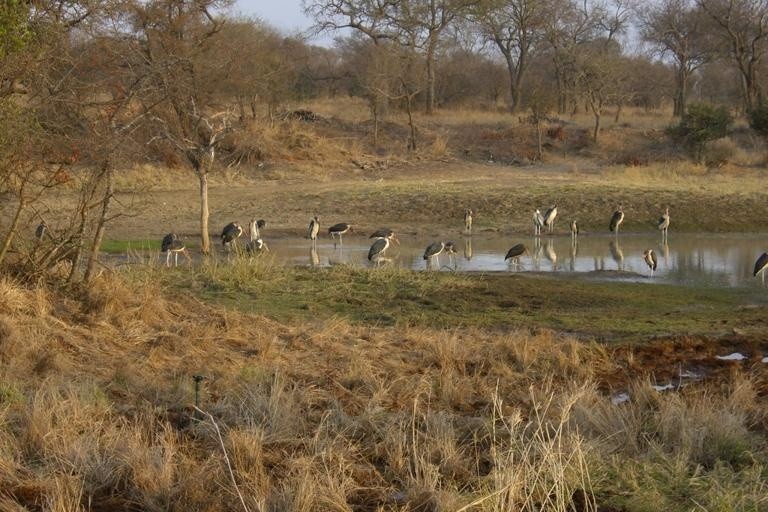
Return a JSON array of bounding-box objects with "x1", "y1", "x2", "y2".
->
[
  {"x1": 753, "y1": 252, "x2": 768, "y2": 284},
  {"x1": 504, "y1": 244, "x2": 530, "y2": 263},
  {"x1": 656, "y1": 208, "x2": 671, "y2": 237},
  {"x1": 308, "y1": 216, "x2": 357, "y2": 244},
  {"x1": 570, "y1": 218, "x2": 580, "y2": 236},
  {"x1": 464, "y1": 208, "x2": 473, "y2": 231},
  {"x1": 220, "y1": 217, "x2": 270, "y2": 260},
  {"x1": 161, "y1": 232, "x2": 192, "y2": 269},
  {"x1": 644, "y1": 247, "x2": 658, "y2": 278},
  {"x1": 368, "y1": 229, "x2": 401, "y2": 266},
  {"x1": 423, "y1": 241, "x2": 454, "y2": 271},
  {"x1": 531, "y1": 204, "x2": 558, "y2": 237},
  {"x1": 609, "y1": 206, "x2": 625, "y2": 232}
]
